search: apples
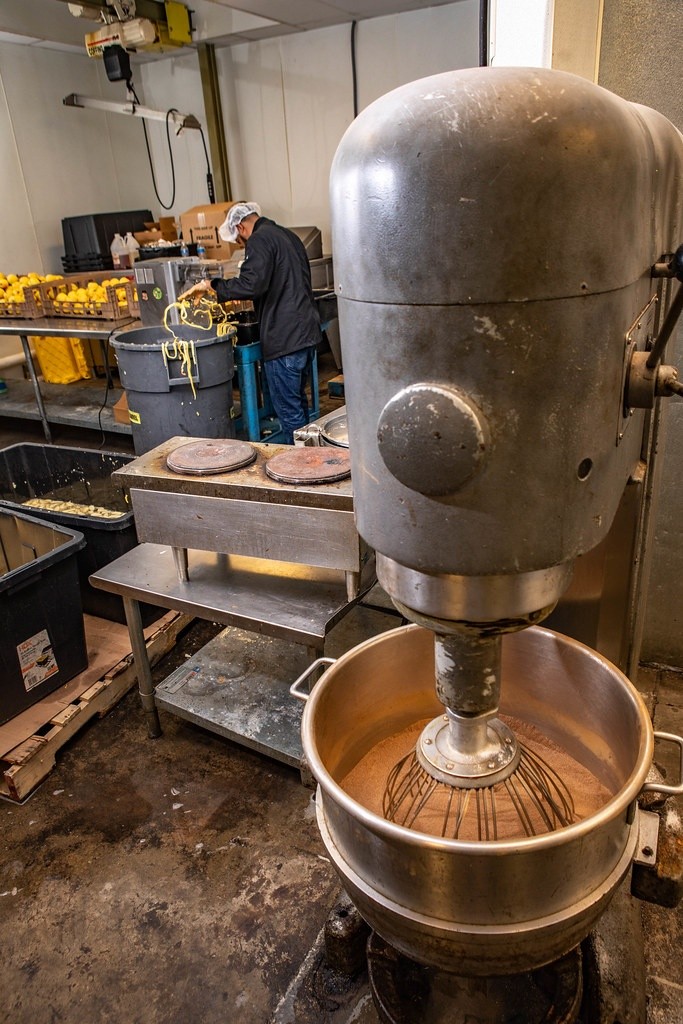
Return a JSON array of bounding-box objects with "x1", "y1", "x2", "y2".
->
[
  {"x1": 54, "y1": 276, "x2": 138, "y2": 314},
  {"x1": 0, "y1": 272, "x2": 78, "y2": 314}
]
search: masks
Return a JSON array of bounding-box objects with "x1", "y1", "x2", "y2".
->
[{"x1": 236, "y1": 233, "x2": 247, "y2": 245}]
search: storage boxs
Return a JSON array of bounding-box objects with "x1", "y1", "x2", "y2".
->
[
  {"x1": 0, "y1": 508, "x2": 89, "y2": 725},
  {"x1": 0, "y1": 201, "x2": 246, "y2": 319},
  {"x1": 0, "y1": 441, "x2": 171, "y2": 629}
]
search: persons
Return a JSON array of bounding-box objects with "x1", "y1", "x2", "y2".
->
[{"x1": 178, "y1": 202, "x2": 322, "y2": 445}]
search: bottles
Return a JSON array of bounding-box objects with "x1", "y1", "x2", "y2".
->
[
  {"x1": 109, "y1": 232, "x2": 141, "y2": 270},
  {"x1": 180, "y1": 242, "x2": 189, "y2": 257},
  {"x1": 197, "y1": 240, "x2": 208, "y2": 259}
]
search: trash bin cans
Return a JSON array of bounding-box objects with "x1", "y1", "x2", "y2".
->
[{"x1": 109, "y1": 322, "x2": 236, "y2": 454}]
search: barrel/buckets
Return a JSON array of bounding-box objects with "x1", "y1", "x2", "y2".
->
[{"x1": 109, "y1": 323, "x2": 238, "y2": 456}]
